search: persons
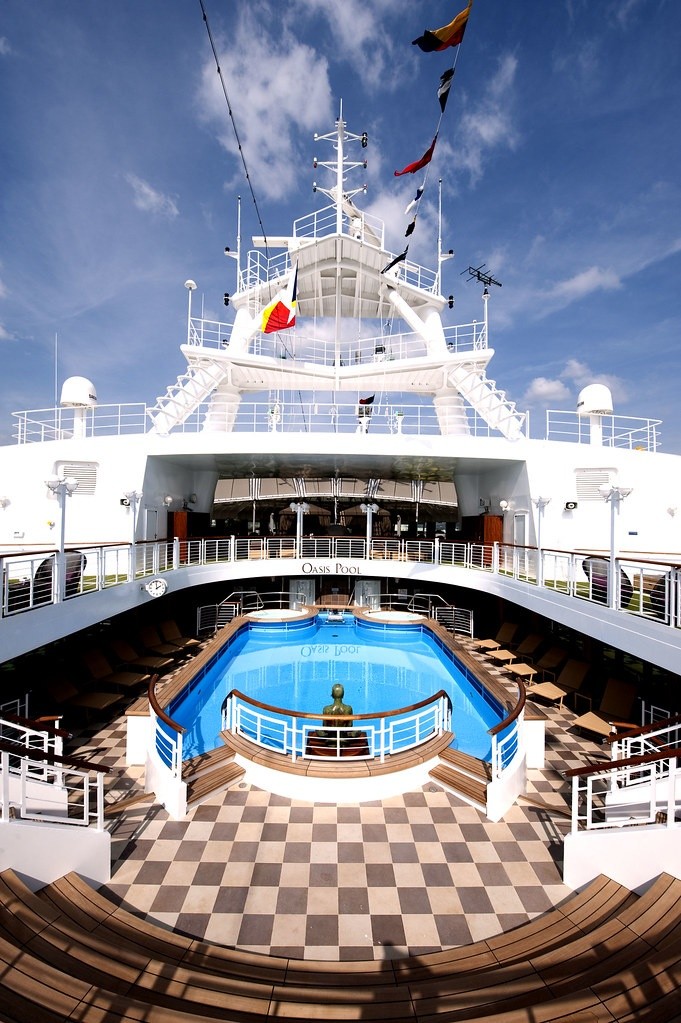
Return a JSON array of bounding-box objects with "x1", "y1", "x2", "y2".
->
[
  {"x1": 329, "y1": 608, "x2": 338, "y2": 615},
  {"x1": 382, "y1": 531, "x2": 392, "y2": 546},
  {"x1": 418, "y1": 532, "x2": 425, "y2": 538},
  {"x1": 333, "y1": 355, "x2": 345, "y2": 366},
  {"x1": 316, "y1": 684, "x2": 361, "y2": 748}
]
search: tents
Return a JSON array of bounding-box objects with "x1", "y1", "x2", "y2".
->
[
  {"x1": 279, "y1": 503, "x2": 331, "y2": 535},
  {"x1": 340, "y1": 504, "x2": 391, "y2": 536}
]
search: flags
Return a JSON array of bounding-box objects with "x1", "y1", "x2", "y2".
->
[
  {"x1": 437, "y1": 68, "x2": 455, "y2": 113},
  {"x1": 381, "y1": 246, "x2": 408, "y2": 274},
  {"x1": 360, "y1": 395, "x2": 374, "y2": 404},
  {"x1": 405, "y1": 186, "x2": 424, "y2": 215},
  {"x1": 412, "y1": 7, "x2": 470, "y2": 53},
  {"x1": 262, "y1": 262, "x2": 299, "y2": 333},
  {"x1": 394, "y1": 132, "x2": 438, "y2": 176},
  {"x1": 405, "y1": 222, "x2": 415, "y2": 237}
]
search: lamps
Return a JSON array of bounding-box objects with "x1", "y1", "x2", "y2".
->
[
  {"x1": 669, "y1": 504, "x2": 679, "y2": 514},
  {"x1": 598, "y1": 475, "x2": 634, "y2": 501},
  {"x1": 0, "y1": 495, "x2": 8, "y2": 509},
  {"x1": 531, "y1": 495, "x2": 552, "y2": 507},
  {"x1": 162, "y1": 496, "x2": 173, "y2": 507},
  {"x1": 360, "y1": 503, "x2": 380, "y2": 513},
  {"x1": 290, "y1": 502, "x2": 311, "y2": 514},
  {"x1": 500, "y1": 500, "x2": 509, "y2": 511},
  {"x1": 123, "y1": 487, "x2": 144, "y2": 500},
  {"x1": 43, "y1": 475, "x2": 80, "y2": 497}
]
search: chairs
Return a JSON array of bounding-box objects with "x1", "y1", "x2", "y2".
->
[
  {"x1": 139, "y1": 625, "x2": 184, "y2": 658},
  {"x1": 484, "y1": 635, "x2": 544, "y2": 666},
  {"x1": 371, "y1": 543, "x2": 427, "y2": 562},
  {"x1": 503, "y1": 646, "x2": 568, "y2": 686},
  {"x1": 42, "y1": 674, "x2": 124, "y2": 721},
  {"x1": 117, "y1": 646, "x2": 175, "y2": 674},
  {"x1": 161, "y1": 617, "x2": 202, "y2": 651},
  {"x1": 575, "y1": 676, "x2": 638, "y2": 738},
  {"x1": 283, "y1": 541, "x2": 294, "y2": 556},
  {"x1": 474, "y1": 623, "x2": 518, "y2": 650},
  {"x1": 651, "y1": 567, "x2": 681, "y2": 620},
  {"x1": 83, "y1": 651, "x2": 151, "y2": 694},
  {"x1": 530, "y1": 659, "x2": 591, "y2": 711},
  {"x1": 582, "y1": 557, "x2": 633, "y2": 607},
  {"x1": 250, "y1": 540, "x2": 267, "y2": 558},
  {"x1": 27, "y1": 551, "x2": 87, "y2": 606}
]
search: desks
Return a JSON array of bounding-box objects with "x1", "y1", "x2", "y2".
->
[{"x1": 8, "y1": 582, "x2": 39, "y2": 614}]
